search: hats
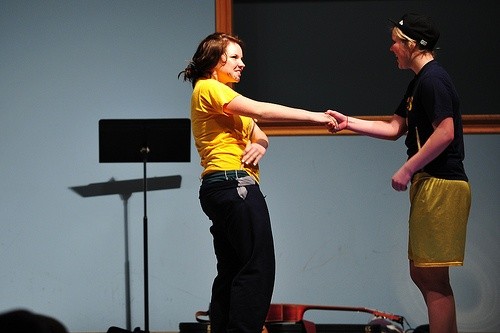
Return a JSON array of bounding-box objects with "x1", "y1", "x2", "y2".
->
[{"x1": 387, "y1": 11, "x2": 440, "y2": 51}]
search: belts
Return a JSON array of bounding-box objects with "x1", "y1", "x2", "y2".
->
[{"x1": 203, "y1": 172, "x2": 247, "y2": 182}]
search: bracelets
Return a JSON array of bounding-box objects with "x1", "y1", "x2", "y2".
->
[{"x1": 346, "y1": 116, "x2": 348, "y2": 129}]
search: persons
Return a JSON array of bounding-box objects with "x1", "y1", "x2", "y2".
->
[
  {"x1": 177, "y1": 33, "x2": 337, "y2": 332},
  {"x1": 325, "y1": 10, "x2": 471, "y2": 333}
]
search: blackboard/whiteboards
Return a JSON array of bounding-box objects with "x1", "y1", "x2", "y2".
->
[{"x1": 214, "y1": 0, "x2": 500, "y2": 136}]
